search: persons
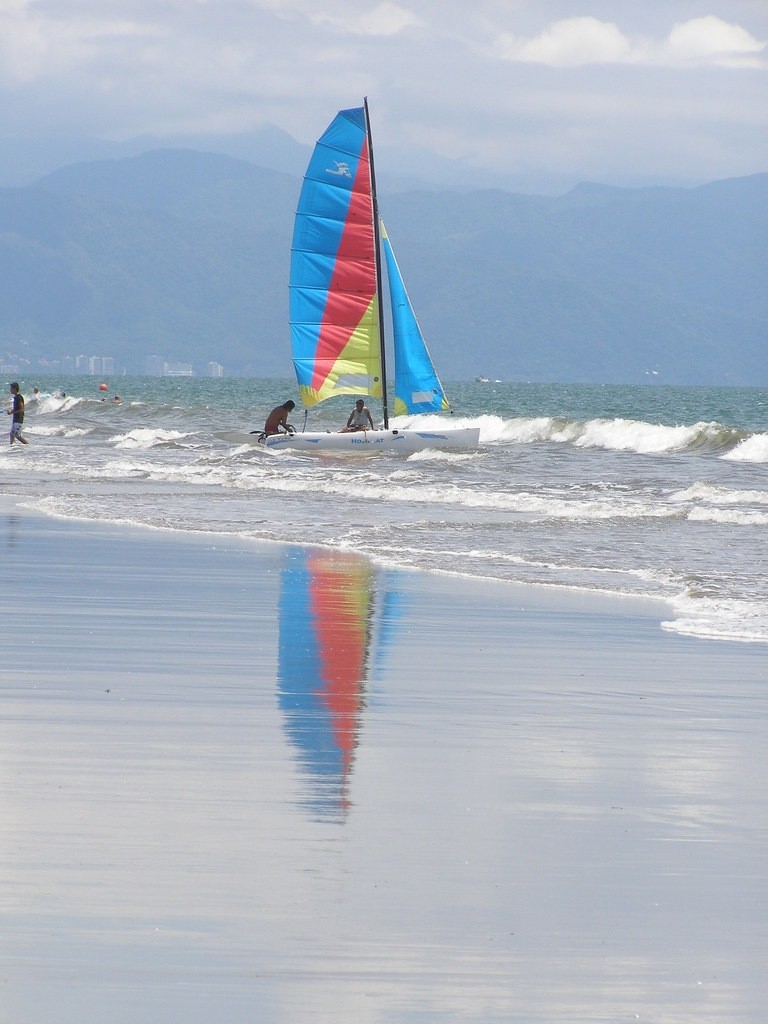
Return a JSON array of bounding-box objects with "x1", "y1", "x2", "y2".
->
[
  {"x1": 327, "y1": 399, "x2": 374, "y2": 434},
  {"x1": 32, "y1": 387, "x2": 40, "y2": 400},
  {"x1": 265, "y1": 400, "x2": 295, "y2": 438},
  {"x1": 4, "y1": 382, "x2": 29, "y2": 445},
  {"x1": 112, "y1": 395, "x2": 123, "y2": 404}
]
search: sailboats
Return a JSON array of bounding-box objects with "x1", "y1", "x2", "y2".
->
[{"x1": 264, "y1": 94, "x2": 482, "y2": 455}]
